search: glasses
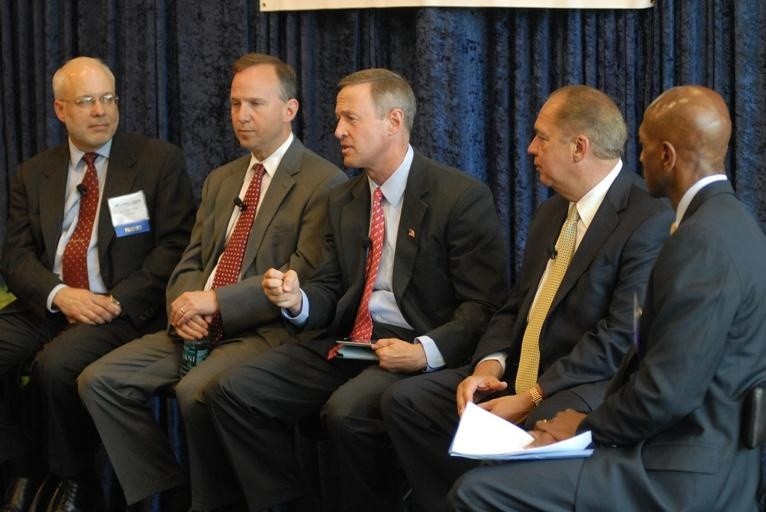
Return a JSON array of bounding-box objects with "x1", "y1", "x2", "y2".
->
[{"x1": 59, "y1": 93, "x2": 120, "y2": 107}]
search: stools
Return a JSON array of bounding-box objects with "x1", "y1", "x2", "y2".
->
[{"x1": 146, "y1": 382, "x2": 186, "y2": 511}]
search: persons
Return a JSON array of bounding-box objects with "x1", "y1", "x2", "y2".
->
[
  {"x1": 446, "y1": 85, "x2": 766, "y2": 512},
  {"x1": 263, "y1": 69, "x2": 511, "y2": 511},
  {"x1": 3, "y1": 56, "x2": 198, "y2": 511},
  {"x1": 380, "y1": 85, "x2": 671, "y2": 511},
  {"x1": 76, "y1": 54, "x2": 352, "y2": 512}
]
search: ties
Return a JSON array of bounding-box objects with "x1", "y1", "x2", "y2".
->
[
  {"x1": 62, "y1": 151, "x2": 100, "y2": 291},
  {"x1": 668, "y1": 222, "x2": 679, "y2": 236},
  {"x1": 328, "y1": 187, "x2": 386, "y2": 361},
  {"x1": 209, "y1": 164, "x2": 267, "y2": 289},
  {"x1": 515, "y1": 202, "x2": 581, "y2": 395}
]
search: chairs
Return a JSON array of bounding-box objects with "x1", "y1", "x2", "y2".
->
[{"x1": 740, "y1": 386, "x2": 766, "y2": 452}]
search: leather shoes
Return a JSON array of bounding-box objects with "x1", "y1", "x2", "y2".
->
[
  {"x1": 48, "y1": 478, "x2": 82, "y2": 512},
  {"x1": 0, "y1": 476, "x2": 30, "y2": 512}
]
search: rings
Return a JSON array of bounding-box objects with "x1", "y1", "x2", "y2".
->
[{"x1": 180, "y1": 308, "x2": 185, "y2": 313}]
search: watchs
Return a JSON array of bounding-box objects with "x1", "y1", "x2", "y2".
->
[{"x1": 528, "y1": 386, "x2": 543, "y2": 408}]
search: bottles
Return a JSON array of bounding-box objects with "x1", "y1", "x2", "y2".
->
[{"x1": 178, "y1": 324, "x2": 210, "y2": 382}]
